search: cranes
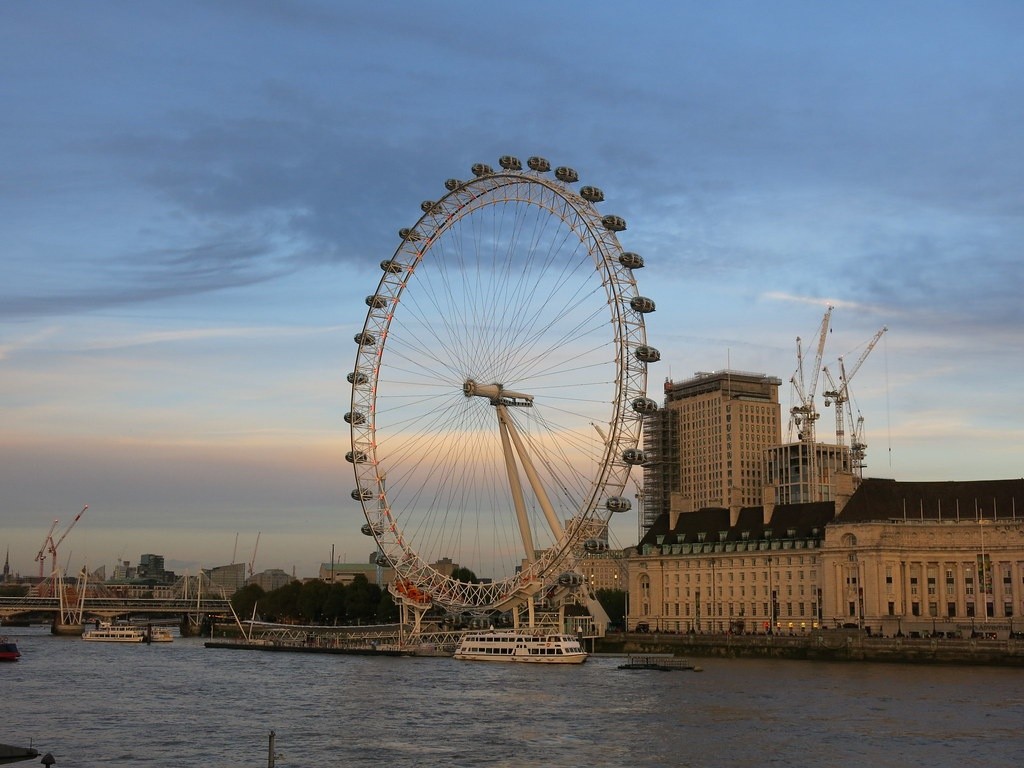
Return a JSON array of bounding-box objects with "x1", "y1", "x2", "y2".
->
[
  {"x1": 591, "y1": 421, "x2": 646, "y2": 545},
  {"x1": 821, "y1": 325, "x2": 889, "y2": 445},
  {"x1": 788, "y1": 306, "x2": 835, "y2": 442},
  {"x1": 838, "y1": 357, "x2": 869, "y2": 480},
  {"x1": 229, "y1": 532, "x2": 240, "y2": 565},
  {"x1": 47, "y1": 504, "x2": 89, "y2": 577},
  {"x1": 62, "y1": 551, "x2": 73, "y2": 578},
  {"x1": 246, "y1": 532, "x2": 261, "y2": 576},
  {"x1": 35, "y1": 519, "x2": 59, "y2": 576}
]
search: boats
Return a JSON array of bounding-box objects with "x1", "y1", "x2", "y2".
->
[
  {"x1": 453, "y1": 633, "x2": 588, "y2": 664},
  {"x1": 204, "y1": 620, "x2": 417, "y2": 656},
  {"x1": 0, "y1": 642, "x2": 22, "y2": 661},
  {"x1": 39, "y1": 626, "x2": 45, "y2": 630},
  {"x1": 81, "y1": 629, "x2": 144, "y2": 642},
  {"x1": 129, "y1": 617, "x2": 151, "y2": 623},
  {"x1": 616, "y1": 652, "x2": 695, "y2": 671},
  {"x1": 99, "y1": 622, "x2": 173, "y2": 643},
  {"x1": 0, "y1": 745, "x2": 56, "y2": 768},
  {"x1": 2, "y1": 620, "x2": 30, "y2": 627},
  {"x1": 86, "y1": 618, "x2": 101, "y2": 624}
]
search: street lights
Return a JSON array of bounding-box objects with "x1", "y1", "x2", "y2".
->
[
  {"x1": 971, "y1": 617, "x2": 977, "y2": 638},
  {"x1": 897, "y1": 615, "x2": 904, "y2": 637},
  {"x1": 833, "y1": 616, "x2": 837, "y2": 629},
  {"x1": 932, "y1": 616, "x2": 937, "y2": 638},
  {"x1": 655, "y1": 614, "x2": 660, "y2": 633},
  {"x1": 1009, "y1": 616, "x2": 1015, "y2": 639},
  {"x1": 729, "y1": 616, "x2": 733, "y2": 635},
  {"x1": 857, "y1": 616, "x2": 861, "y2": 628},
  {"x1": 768, "y1": 616, "x2": 772, "y2": 635},
  {"x1": 691, "y1": 615, "x2": 696, "y2": 634},
  {"x1": 810, "y1": 616, "x2": 814, "y2": 635}
]
